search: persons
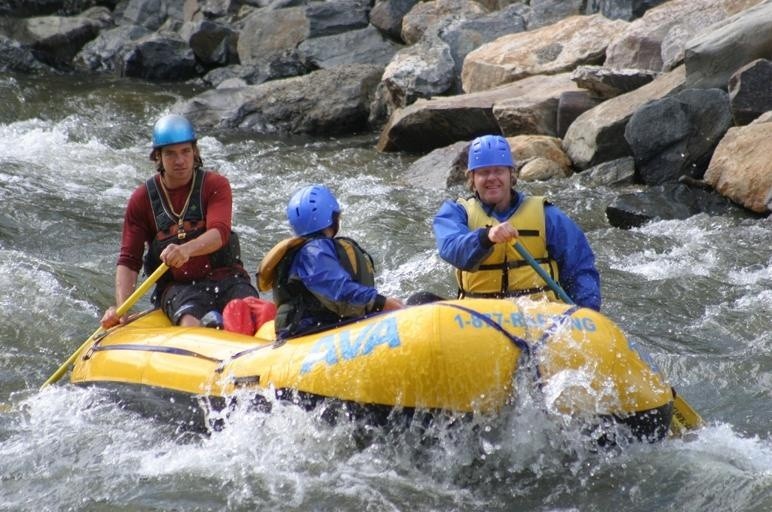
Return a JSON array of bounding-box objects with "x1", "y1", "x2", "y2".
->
[
  {"x1": 254, "y1": 184, "x2": 403, "y2": 344},
  {"x1": 99, "y1": 113, "x2": 275, "y2": 339},
  {"x1": 407, "y1": 133, "x2": 601, "y2": 313}
]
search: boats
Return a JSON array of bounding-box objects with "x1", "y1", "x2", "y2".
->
[{"x1": 49, "y1": 293, "x2": 678, "y2": 454}]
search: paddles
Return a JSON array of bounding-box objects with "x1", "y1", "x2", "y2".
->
[
  {"x1": 38, "y1": 263, "x2": 169, "y2": 393},
  {"x1": 508, "y1": 239, "x2": 707, "y2": 436}
]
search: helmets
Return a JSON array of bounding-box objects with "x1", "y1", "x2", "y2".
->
[
  {"x1": 467, "y1": 136, "x2": 514, "y2": 171},
  {"x1": 153, "y1": 116, "x2": 195, "y2": 148},
  {"x1": 288, "y1": 186, "x2": 339, "y2": 236}
]
